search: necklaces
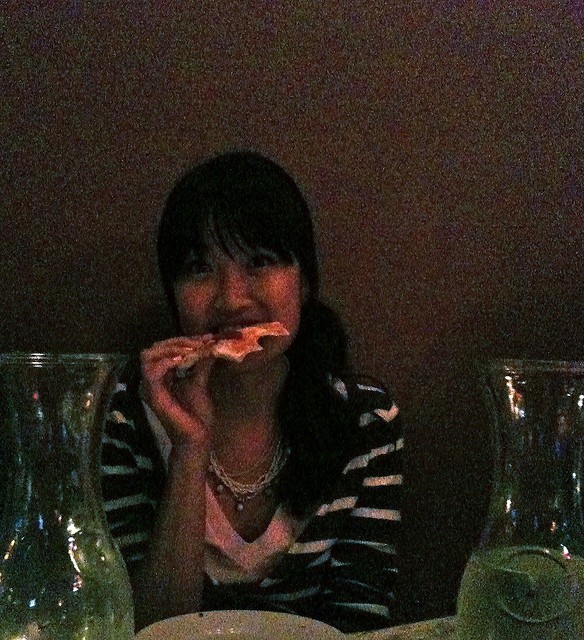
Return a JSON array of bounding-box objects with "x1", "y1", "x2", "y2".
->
[{"x1": 206, "y1": 429, "x2": 290, "y2": 511}]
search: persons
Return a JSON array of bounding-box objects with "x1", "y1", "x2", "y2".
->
[{"x1": 99, "y1": 150, "x2": 407, "y2": 632}]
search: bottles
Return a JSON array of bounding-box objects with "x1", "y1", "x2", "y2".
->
[
  {"x1": 0, "y1": 350, "x2": 137, "y2": 639},
  {"x1": 454, "y1": 357, "x2": 584, "y2": 639}
]
